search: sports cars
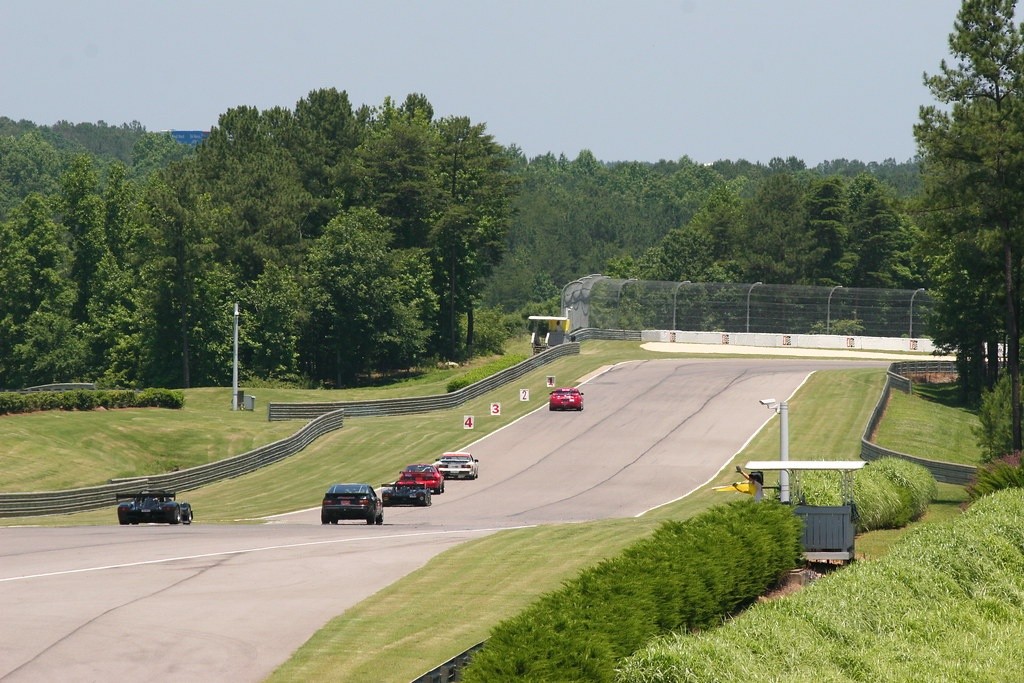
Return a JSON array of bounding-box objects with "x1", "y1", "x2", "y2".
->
[
  {"x1": 380, "y1": 481, "x2": 433, "y2": 507},
  {"x1": 115, "y1": 489, "x2": 193, "y2": 525}
]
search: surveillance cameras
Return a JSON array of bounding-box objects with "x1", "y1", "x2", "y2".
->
[{"x1": 758, "y1": 399, "x2": 776, "y2": 405}]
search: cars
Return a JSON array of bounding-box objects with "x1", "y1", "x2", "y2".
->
[
  {"x1": 400, "y1": 464, "x2": 446, "y2": 495},
  {"x1": 435, "y1": 452, "x2": 479, "y2": 480},
  {"x1": 550, "y1": 388, "x2": 585, "y2": 410},
  {"x1": 321, "y1": 483, "x2": 384, "y2": 525}
]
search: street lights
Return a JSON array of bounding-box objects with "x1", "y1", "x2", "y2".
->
[
  {"x1": 827, "y1": 286, "x2": 843, "y2": 334},
  {"x1": 615, "y1": 279, "x2": 638, "y2": 328},
  {"x1": 746, "y1": 282, "x2": 763, "y2": 332},
  {"x1": 910, "y1": 288, "x2": 925, "y2": 336},
  {"x1": 673, "y1": 281, "x2": 691, "y2": 330}
]
